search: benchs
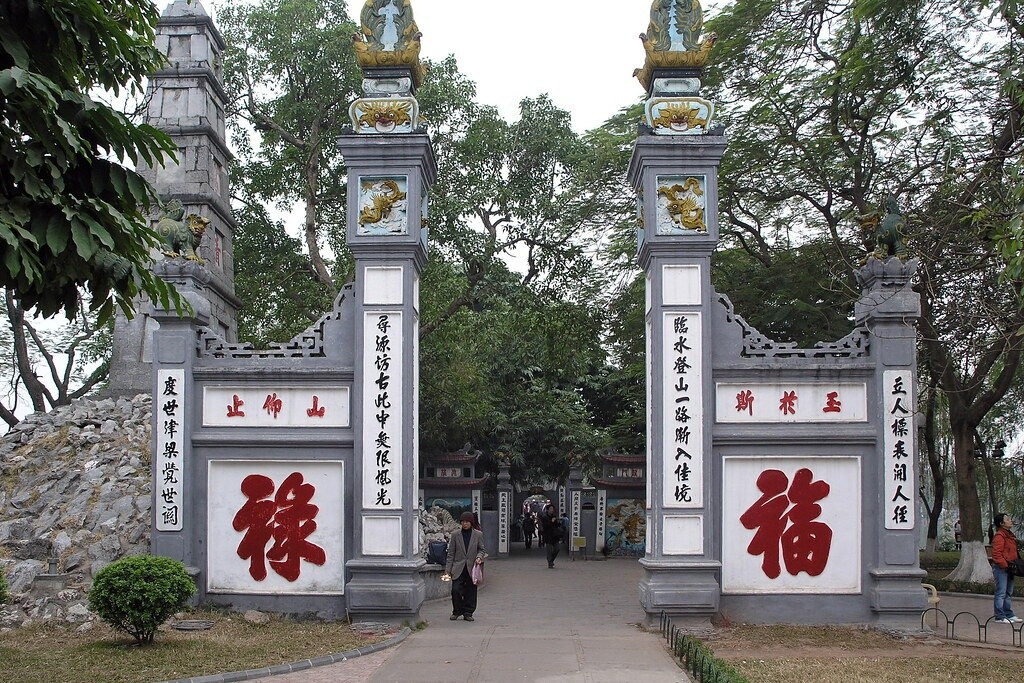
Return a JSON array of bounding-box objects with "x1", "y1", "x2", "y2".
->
[{"x1": 954, "y1": 530, "x2": 962, "y2": 550}]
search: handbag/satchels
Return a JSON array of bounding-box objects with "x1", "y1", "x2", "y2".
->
[
  {"x1": 471, "y1": 562, "x2": 482, "y2": 585},
  {"x1": 1006, "y1": 558, "x2": 1024, "y2": 577}
]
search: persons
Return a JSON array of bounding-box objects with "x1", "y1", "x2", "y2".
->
[
  {"x1": 542, "y1": 504, "x2": 560, "y2": 568},
  {"x1": 988, "y1": 524, "x2": 994, "y2": 545},
  {"x1": 522, "y1": 513, "x2": 535, "y2": 550},
  {"x1": 535, "y1": 515, "x2": 545, "y2": 548},
  {"x1": 991, "y1": 512, "x2": 1023, "y2": 624},
  {"x1": 562, "y1": 513, "x2": 569, "y2": 543},
  {"x1": 955, "y1": 520, "x2": 962, "y2": 550},
  {"x1": 445, "y1": 512, "x2": 485, "y2": 621},
  {"x1": 523, "y1": 504, "x2": 537, "y2": 538}
]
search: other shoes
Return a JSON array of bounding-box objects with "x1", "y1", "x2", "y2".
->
[
  {"x1": 995, "y1": 617, "x2": 1014, "y2": 623},
  {"x1": 464, "y1": 616, "x2": 474, "y2": 621},
  {"x1": 548, "y1": 563, "x2": 554, "y2": 568},
  {"x1": 1007, "y1": 616, "x2": 1023, "y2": 623},
  {"x1": 450, "y1": 614, "x2": 458, "y2": 620}
]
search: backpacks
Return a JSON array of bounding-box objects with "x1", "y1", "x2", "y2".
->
[{"x1": 428, "y1": 542, "x2": 447, "y2": 565}]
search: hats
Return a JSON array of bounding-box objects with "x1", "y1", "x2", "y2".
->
[{"x1": 459, "y1": 511, "x2": 474, "y2": 526}]
